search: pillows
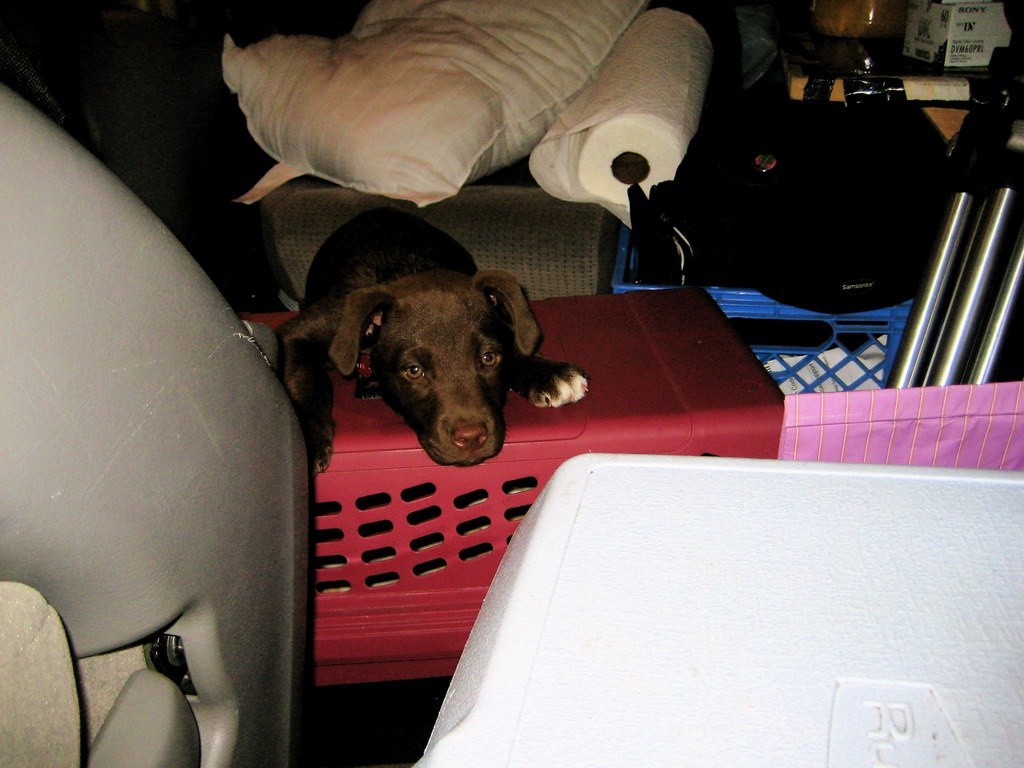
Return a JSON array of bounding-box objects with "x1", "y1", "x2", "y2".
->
[{"x1": 220, "y1": 0, "x2": 649, "y2": 207}]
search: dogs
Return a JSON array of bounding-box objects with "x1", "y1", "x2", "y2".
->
[{"x1": 277, "y1": 207, "x2": 590, "y2": 477}]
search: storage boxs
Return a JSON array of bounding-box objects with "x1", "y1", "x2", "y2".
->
[
  {"x1": 902, "y1": 1, "x2": 1011, "y2": 68},
  {"x1": 610, "y1": 225, "x2": 916, "y2": 395}
]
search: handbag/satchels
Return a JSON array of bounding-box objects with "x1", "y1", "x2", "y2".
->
[
  {"x1": 708, "y1": 98, "x2": 971, "y2": 316},
  {"x1": 624, "y1": 183, "x2": 764, "y2": 287}
]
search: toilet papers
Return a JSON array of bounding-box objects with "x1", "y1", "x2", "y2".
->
[{"x1": 529, "y1": 7, "x2": 710, "y2": 229}]
